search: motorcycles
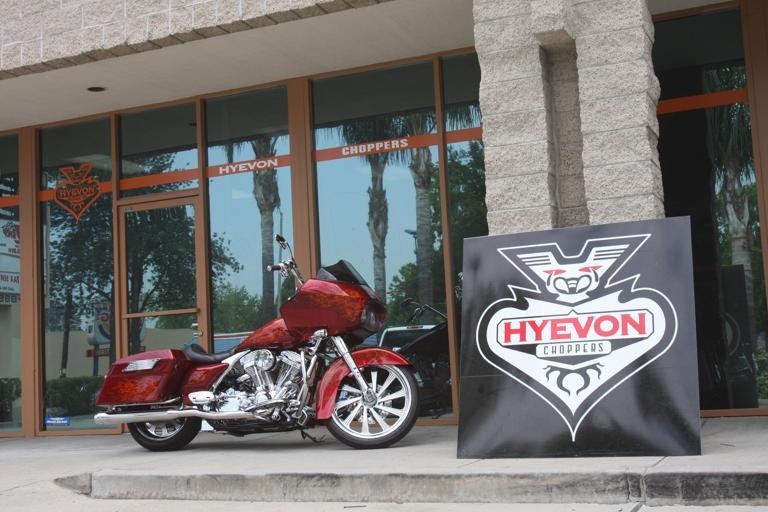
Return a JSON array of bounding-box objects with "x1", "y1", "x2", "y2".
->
[
  {"x1": 386, "y1": 270, "x2": 462, "y2": 421},
  {"x1": 95, "y1": 235, "x2": 422, "y2": 449}
]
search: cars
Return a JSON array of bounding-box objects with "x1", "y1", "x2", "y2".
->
[{"x1": 379, "y1": 326, "x2": 436, "y2": 385}]
